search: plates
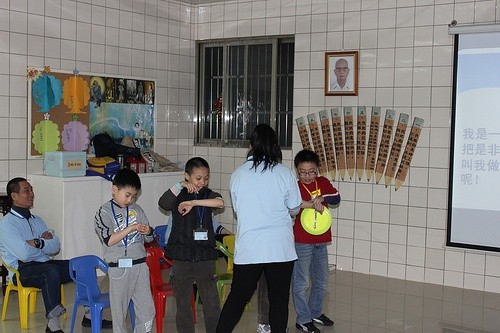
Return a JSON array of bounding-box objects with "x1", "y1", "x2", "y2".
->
[{"x1": 300, "y1": 205, "x2": 332, "y2": 235}]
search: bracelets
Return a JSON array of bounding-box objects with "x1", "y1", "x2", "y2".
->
[
  {"x1": 147, "y1": 226, "x2": 153, "y2": 236},
  {"x1": 175, "y1": 182, "x2": 184, "y2": 190}
]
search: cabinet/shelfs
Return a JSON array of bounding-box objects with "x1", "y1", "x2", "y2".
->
[{"x1": 24, "y1": 171, "x2": 185, "y2": 276}]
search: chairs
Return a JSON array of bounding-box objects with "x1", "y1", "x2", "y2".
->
[{"x1": 1, "y1": 224, "x2": 248, "y2": 332}]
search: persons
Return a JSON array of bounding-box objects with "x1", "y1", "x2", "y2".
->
[
  {"x1": 158, "y1": 156, "x2": 225, "y2": 333},
  {"x1": 133, "y1": 122, "x2": 148, "y2": 147},
  {"x1": 0, "y1": 177, "x2": 113, "y2": 333},
  {"x1": 329, "y1": 58, "x2": 353, "y2": 92},
  {"x1": 93, "y1": 167, "x2": 156, "y2": 333},
  {"x1": 214, "y1": 122, "x2": 303, "y2": 333},
  {"x1": 256, "y1": 270, "x2": 290, "y2": 333},
  {"x1": 290, "y1": 148, "x2": 341, "y2": 333}
]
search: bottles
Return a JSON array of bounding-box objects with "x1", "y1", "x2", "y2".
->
[{"x1": 117, "y1": 154, "x2": 124, "y2": 169}]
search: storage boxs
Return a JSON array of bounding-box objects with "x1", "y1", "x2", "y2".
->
[{"x1": 44, "y1": 151, "x2": 87, "y2": 178}]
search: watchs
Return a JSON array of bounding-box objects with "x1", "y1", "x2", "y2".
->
[{"x1": 33, "y1": 238, "x2": 40, "y2": 248}]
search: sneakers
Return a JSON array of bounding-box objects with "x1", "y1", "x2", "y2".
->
[
  {"x1": 296, "y1": 322, "x2": 320, "y2": 333},
  {"x1": 312, "y1": 313, "x2": 334, "y2": 326}
]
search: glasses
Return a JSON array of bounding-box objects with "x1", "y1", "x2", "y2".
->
[
  {"x1": 335, "y1": 67, "x2": 348, "y2": 72},
  {"x1": 297, "y1": 168, "x2": 317, "y2": 176}
]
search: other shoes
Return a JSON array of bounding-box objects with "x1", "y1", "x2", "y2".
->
[
  {"x1": 46, "y1": 324, "x2": 63, "y2": 333},
  {"x1": 82, "y1": 315, "x2": 112, "y2": 327},
  {"x1": 257, "y1": 323, "x2": 271, "y2": 333}
]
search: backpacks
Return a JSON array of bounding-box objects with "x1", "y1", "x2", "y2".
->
[
  {"x1": 85, "y1": 156, "x2": 120, "y2": 180},
  {"x1": 92, "y1": 134, "x2": 117, "y2": 160}
]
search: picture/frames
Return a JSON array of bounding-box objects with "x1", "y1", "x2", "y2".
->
[{"x1": 324, "y1": 52, "x2": 359, "y2": 96}]
